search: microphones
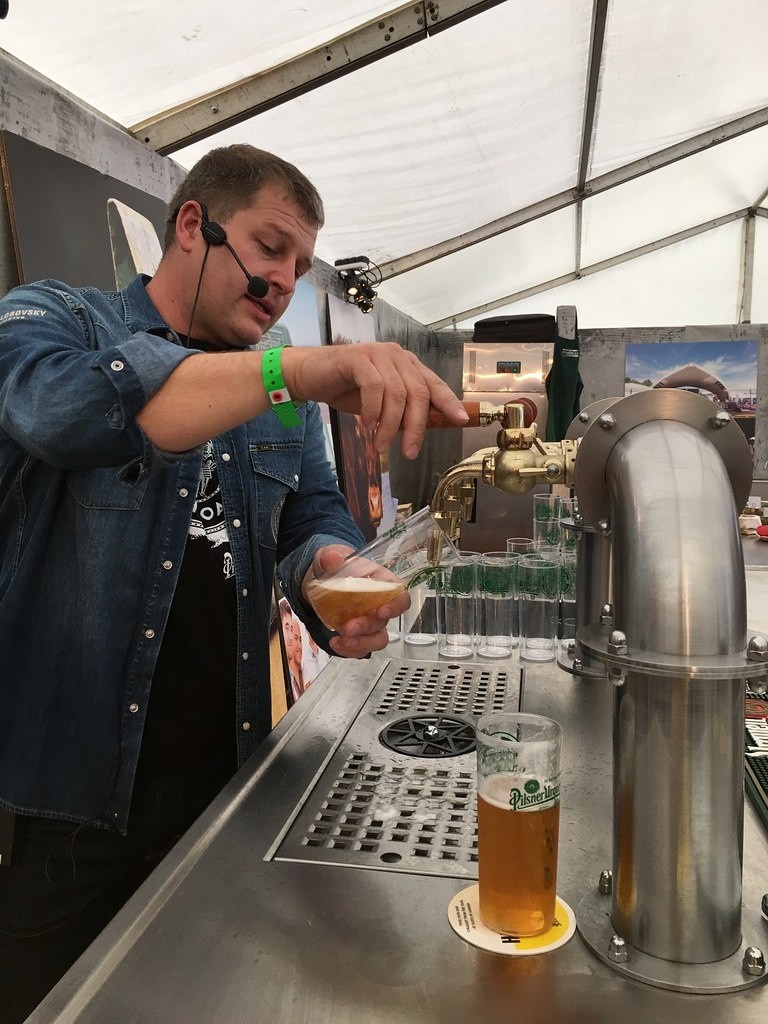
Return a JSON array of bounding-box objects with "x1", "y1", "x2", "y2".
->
[{"x1": 200, "y1": 220, "x2": 269, "y2": 297}]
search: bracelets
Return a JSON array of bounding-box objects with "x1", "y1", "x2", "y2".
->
[{"x1": 262, "y1": 344, "x2": 305, "y2": 433}]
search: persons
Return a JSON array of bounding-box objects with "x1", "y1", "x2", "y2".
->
[
  {"x1": 288, "y1": 618, "x2": 307, "y2": 697},
  {"x1": 299, "y1": 634, "x2": 330, "y2": 688},
  {"x1": 2, "y1": 142, "x2": 470, "y2": 1023},
  {"x1": 279, "y1": 599, "x2": 297, "y2": 707}
]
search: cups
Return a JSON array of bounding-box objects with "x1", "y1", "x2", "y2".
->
[
  {"x1": 436, "y1": 493, "x2": 579, "y2": 662},
  {"x1": 303, "y1": 505, "x2": 464, "y2": 631},
  {"x1": 386, "y1": 614, "x2": 404, "y2": 641},
  {"x1": 474, "y1": 712, "x2": 564, "y2": 936},
  {"x1": 401, "y1": 574, "x2": 438, "y2": 646}
]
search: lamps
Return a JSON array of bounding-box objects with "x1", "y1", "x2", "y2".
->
[{"x1": 334, "y1": 254, "x2": 384, "y2": 314}]
